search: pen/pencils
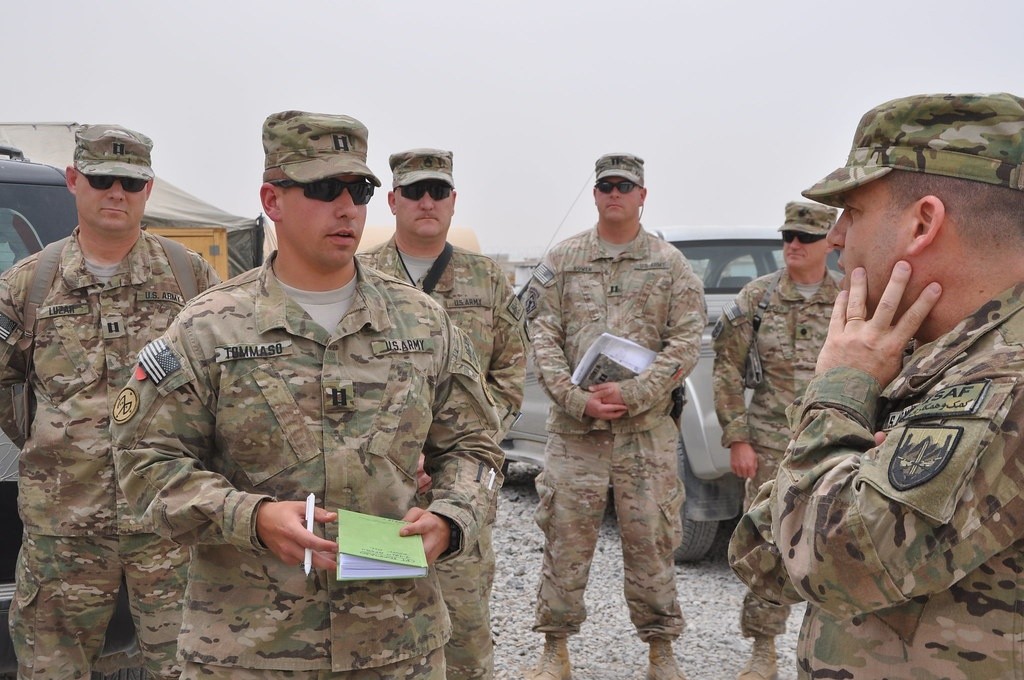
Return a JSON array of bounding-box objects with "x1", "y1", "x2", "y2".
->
[
  {"x1": 670, "y1": 364, "x2": 681, "y2": 377},
  {"x1": 511, "y1": 411, "x2": 522, "y2": 430},
  {"x1": 673, "y1": 368, "x2": 684, "y2": 380},
  {"x1": 304, "y1": 493, "x2": 315, "y2": 576},
  {"x1": 488, "y1": 468, "x2": 495, "y2": 490},
  {"x1": 476, "y1": 462, "x2": 486, "y2": 481}
]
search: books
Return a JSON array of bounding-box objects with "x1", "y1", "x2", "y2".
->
[
  {"x1": 334, "y1": 506, "x2": 429, "y2": 582},
  {"x1": 579, "y1": 353, "x2": 638, "y2": 392}
]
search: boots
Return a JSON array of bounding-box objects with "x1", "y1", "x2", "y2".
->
[
  {"x1": 645, "y1": 637, "x2": 687, "y2": 680},
  {"x1": 739, "y1": 636, "x2": 777, "y2": 680},
  {"x1": 532, "y1": 635, "x2": 573, "y2": 680}
]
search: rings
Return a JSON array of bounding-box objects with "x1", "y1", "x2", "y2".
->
[{"x1": 847, "y1": 316, "x2": 866, "y2": 322}]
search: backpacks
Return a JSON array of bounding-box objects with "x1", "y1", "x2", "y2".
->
[{"x1": 12, "y1": 236, "x2": 199, "y2": 441}]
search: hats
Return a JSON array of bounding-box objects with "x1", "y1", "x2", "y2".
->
[
  {"x1": 388, "y1": 148, "x2": 455, "y2": 189},
  {"x1": 802, "y1": 93, "x2": 1024, "y2": 208},
  {"x1": 262, "y1": 110, "x2": 381, "y2": 198},
  {"x1": 595, "y1": 152, "x2": 644, "y2": 187},
  {"x1": 777, "y1": 201, "x2": 838, "y2": 236},
  {"x1": 73, "y1": 123, "x2": 155, "y2": 181}
]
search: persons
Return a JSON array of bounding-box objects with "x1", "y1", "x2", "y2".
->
[
  {"x1": 524, "y1": 153, "x2": 706, "y2": 680},
  {"x1": 0, "y1": 126, "x2": 220, "y2": 679},
  {"x1": 353, "y1": 148, "x2": 523, "y2": 680},
  {"x1": 770, "y1": 87, "x2": 1023, "y2": 680},
  {"x1": 106, "y1": 110, "x2": 505, "y2": 680},
  {"x1": 710, "y1": 200, "x2": 844, "y2": 680}
]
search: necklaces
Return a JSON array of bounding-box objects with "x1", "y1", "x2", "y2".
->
[{"x1": 394, "y1": 241, "x2": 418, "y2": 289}]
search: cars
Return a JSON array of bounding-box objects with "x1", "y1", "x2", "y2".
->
[{"x1": 0, "y1": 144, "x2": 80, "y2": 619}]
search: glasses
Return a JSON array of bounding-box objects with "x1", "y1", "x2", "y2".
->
[
  {"x1": 76, "y1": 168, "x2": 147, "y2": 192},
  {"x1": 782, "y1": 230, "x2": 827, "y2": 244},
  {"x1": 399, "y1": 180, "x2": 453, "y2": 200},
  {"x1": 595, "y1": 180, "x2": 637, "y2": 194},
  {"x1": 272, "y1": 177, "x2": 374, "y2": 205}
]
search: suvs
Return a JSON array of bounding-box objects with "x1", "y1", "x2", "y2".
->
[{"x1": 498, "y1": 226, "x2": 788, "y2": 569}]
